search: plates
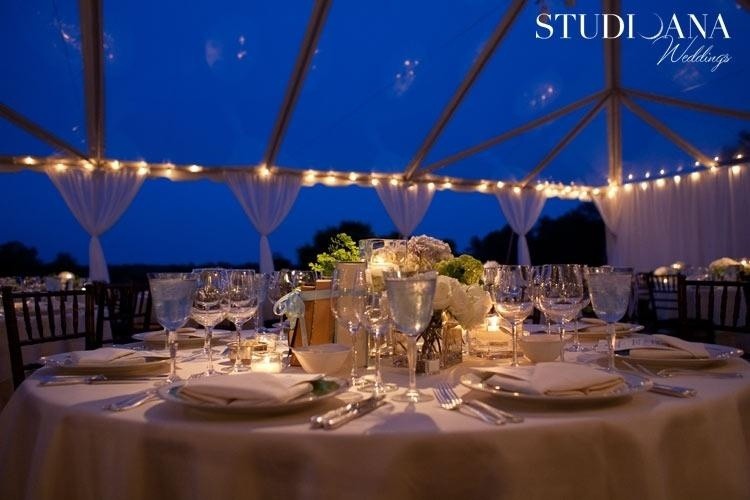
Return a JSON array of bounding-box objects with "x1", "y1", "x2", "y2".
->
[
  {"x1": 548, "y1": 324, "x2": 645, "y2": 339},
  {"x1": 133, "y1": 329, "x2": 231, "y2": 346},
  {"x1": 609, "y1": 345, "x2": 734, "y2": 368},
  {"x1": 460, "y1": 365, "x2": 628, "y2": 405},
  {"x1": 40, "y1": 353, "x2": 168, "y2": 378},
  {"x1": 159, "y1": 380, "x2": 339, "y2": 404}
]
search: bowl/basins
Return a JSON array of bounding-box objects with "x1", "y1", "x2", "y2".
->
[
  {"x1": 293, "y1": 343, "x2": 352, "y2": 377},
  {"x1": 517, "y1": 334, "x2": 573, "y2": 362}
]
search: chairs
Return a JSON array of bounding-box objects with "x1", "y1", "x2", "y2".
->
[
  {"x1": 0, "y1": 271, "x2": 153, "y2": 386},
  {"x1": 516, "y1": 264, "x2": 749, "y2": 357}
]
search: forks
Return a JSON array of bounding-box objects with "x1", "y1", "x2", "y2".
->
[{"x1": 426, "y1": 375, "x2": 524, "y2": 429}]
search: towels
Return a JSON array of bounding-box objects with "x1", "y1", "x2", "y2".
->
[
  {"x1": 468, "y1": 363, "x2": 624, "y2": 397},
  {"x1": 575, "y1": 318, "x2": 632, "y2": 334},
  {"x1": 144, "y1": 327, "x2": 216, "y2": 340},
  {"x1": 178, "y1": 372, "x2": 324, "y2": 407},
  {"x1": 629, "y1": 334, "x2": 709, "y2": 359},
  {"x1": 65, "y1": 345, "x2": 146, "y2": 366}
]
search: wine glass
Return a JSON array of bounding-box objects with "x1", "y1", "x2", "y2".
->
[
  {"x1": 526, "y1": 264, "x2": 587, "y2": 365},
  {"x1": 383, "y1": 276, "x2": 438, "y2": 404},
  {"x1": 329, "y1": 266, "x2": 400, "y2": 396},
  {"x1": 150, "y1": 270, "x2": 195, "y2": 390},
  {"x1": 216, "y1": 269, "x2": 262, "y2": 373},
  {"x1": 1, "y1": 275, "x2": 42, "y2": 308},
  {"x1": 189, "y1": 269, "x2": 228, "y2": 381},
  {"x1": 584, "y1": 264, "x2": 633, "y2": 356},
  {"x1": 484, "y1": 264, "x2": 531, "y2": 368},
  {"x1": 267, "y1": 267, "x2": 313, "y2": 341}
]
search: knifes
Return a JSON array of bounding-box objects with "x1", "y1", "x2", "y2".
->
[
  {"x1": 307, "y1": 391, "x2": 386, "y2": 430},
  {"x1": 620, "y1": 370, "x2": 698, "y2": 399}
]
